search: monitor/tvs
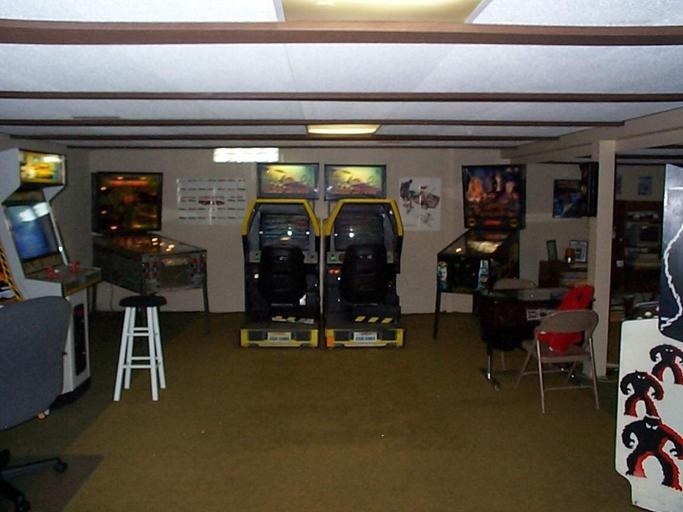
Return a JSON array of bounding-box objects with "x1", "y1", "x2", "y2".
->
[
  {"x1": 4, "y1": 203, "x2": 53, "y2": 262},
  {"x1": 334, "y1": 212, "x2": 385, "y2": 252},
  {"x1": 258, "y1": 212, "x2": 310, "y2": 251}
]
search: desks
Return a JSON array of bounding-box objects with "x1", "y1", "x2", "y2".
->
[
  {"x1": 538, "y1": 257, "x2": 664, "y2": 294},
  {"x1": 476, "y1": 287, "x2": 590, "y2": 392},
  {"x1": 432, "y1": 164, "x2": 527, "y2": 341},
  {"x1": 91, "y1": 170, "x2": 209, "y2": 357}
]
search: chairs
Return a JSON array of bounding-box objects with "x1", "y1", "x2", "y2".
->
[
  {"x1": 0, "y1": 294, "x2": 75, "y2": 512},
  {"x1": 514, "y1": 309, "x2": 603, "y2": 414}
]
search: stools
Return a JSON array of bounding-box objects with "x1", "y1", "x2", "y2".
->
[{"x1": 113, "y1": 295, "x2": 166, "y2": 402}]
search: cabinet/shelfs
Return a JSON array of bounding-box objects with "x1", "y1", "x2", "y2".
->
[{"x1": 615, "y1": 162, "x2": 683, "y2": 512}]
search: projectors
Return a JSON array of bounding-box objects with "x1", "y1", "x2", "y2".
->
[{"x1": 517, "y1": 288, "x2": 552, "y2": 302}]
